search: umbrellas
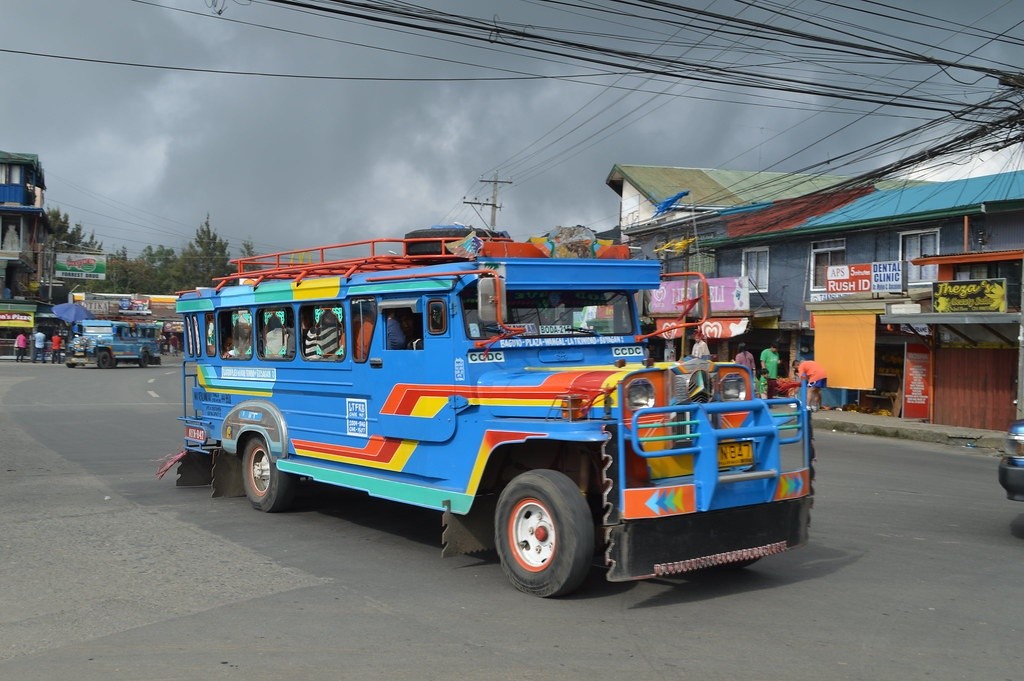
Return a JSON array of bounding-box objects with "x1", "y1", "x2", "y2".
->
[{"x1": 50, "y1": 303, "x2": 96, "y2": 323}]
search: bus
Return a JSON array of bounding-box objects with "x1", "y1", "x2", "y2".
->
[
  {"x1": 154, "y1": 225, "x2": 815, "y2": 600},
  {"x1": 65, "y1": 320, "x2": 162, "y2": 369}
]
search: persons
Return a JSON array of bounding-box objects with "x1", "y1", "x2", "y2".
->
[
  {"x1": 386, "y1": 308, "x2": 406, "y2": 350},
  {"x1": 158, "y1": 332, "x2": 166, "y2": 354},
  {"x1": 32, "y1": 326, "x2": 46, "y2": 363},
  {"x1": 266, "y1": 310, "x2": 284, "y2": 331},
  {"x1": 692, "y1": 332, "x2": 710, "y2": 360},
  {"x1": 16, "y1": 329, "x2": 26, "y2": 362},
  {"x1": 168, "y1": 332, "x2": 179, "y2": 357},
  {"x1": 792, "y1": 359, "x2": 827, "y2": 413},
  {"x1": 52, "y1": 331, "x2": 61, "y2": 364},
  {"x1": 352, "y1": 303, "x2": 375, "y2": 360},
  {"x1": 317, "y1": 305, "x2": 341, "y2": 328},
  {"x1": 760, "y1": 369, "x2": 769, "y2": 398},
  {"x1": 734, "y1": 342, "x2": 756, "y2": 378},
  {"x1": 760, "y1": 342, "x2": 782, "y2": 399}
]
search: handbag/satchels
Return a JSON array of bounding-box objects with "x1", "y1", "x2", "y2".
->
[{"x1": 14, "y1": 337, "x2": 19, "y2": 347}]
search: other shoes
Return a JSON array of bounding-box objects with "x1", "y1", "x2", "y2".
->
[{"x1": 806, "y1": 405, "x2": 817, "y2": 412}]
